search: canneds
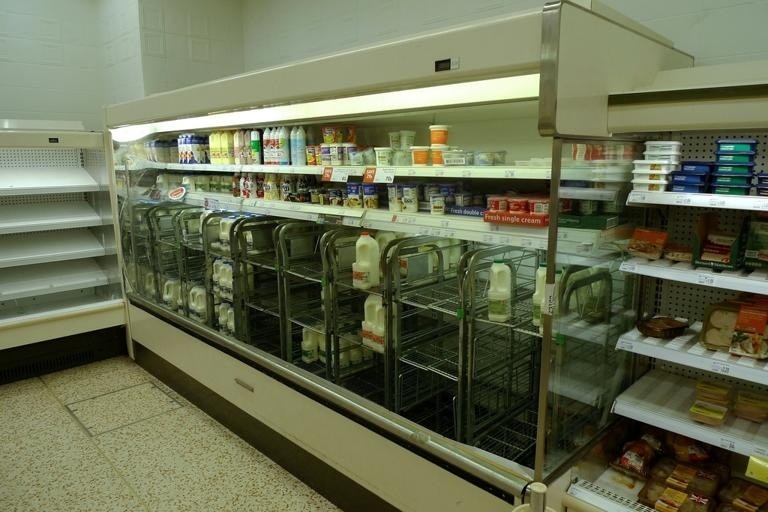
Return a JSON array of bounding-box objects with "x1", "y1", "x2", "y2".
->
[{"x1": 347, "y1": 181, "x2": 378, "y2": 208}]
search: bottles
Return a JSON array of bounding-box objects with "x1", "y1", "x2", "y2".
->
[
  {"x1": 178, "y1": 173, "x2": 316, "y2": 203},
  {"x1": 144, "y1": 136, "x2": 178, "y2": 164},
  {"x1": 177, "y1": 125, "x2": 314, "y2": 165}
]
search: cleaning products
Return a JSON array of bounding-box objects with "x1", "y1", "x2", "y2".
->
[{"x1": 207, "y1": 129, "x2": 307, "y2": 165}]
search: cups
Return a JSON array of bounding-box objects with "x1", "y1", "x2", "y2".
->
[{"x1": 306, "y1": 123, "x2": 512, "y2": 166}]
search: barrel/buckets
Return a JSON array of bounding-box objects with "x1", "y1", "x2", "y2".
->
[
  {"x1": 488, "y1": 259, "x2": 512, "y2": 322},
  {"x1": 144, "y1": 212, "x2": 266, "y2": 338},
  {"x1": 301, "y1": 317, "x2": 374, "y2": 368},
  {"x1": 533, "y1": 263, "x2": 547, "y2": 326},
  {"x1": 353, "y1": 231, "x2": 380, "y2": 289},
  {"x1": 362, "y1": 294, "x2": 387, "y2": 352}
]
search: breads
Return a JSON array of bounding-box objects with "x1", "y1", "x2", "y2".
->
[
  {"x1": 691, "y1": 378, "x2": 767, "y2": 425},
  {"x1": 621, "y1": 429, "x2": 768, "y2": 512}
]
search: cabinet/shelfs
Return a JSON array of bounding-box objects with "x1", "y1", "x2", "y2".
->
[
  {"x1": 99, "y1": 1, "x2": 702, "y2": 512},
  {"x1": 0, "y1": 117, "x2": 136, "y2": 387},
  {"x1": 512, "y1": 56, "x2": 768, "y2": 512}
]
